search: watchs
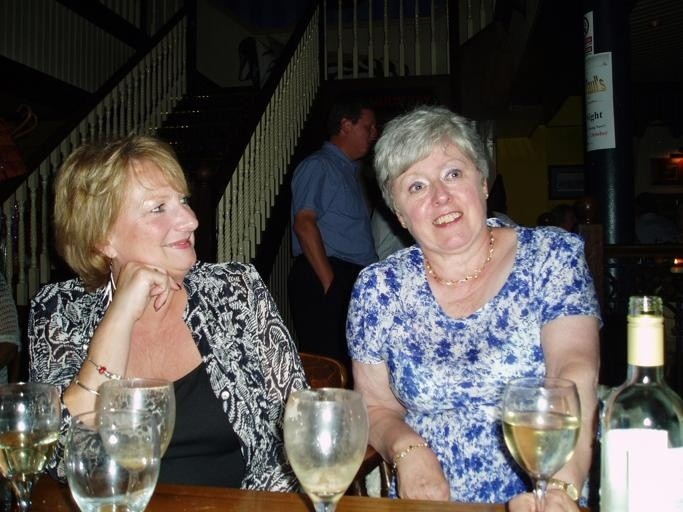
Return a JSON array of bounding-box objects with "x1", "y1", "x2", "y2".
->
[{"x1": 545, "y1": 477, "x2": 580, "y2": 503}]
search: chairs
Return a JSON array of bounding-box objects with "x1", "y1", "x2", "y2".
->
[{"x1": 298, "y1": 352, "x2": 400, "y2": 496}]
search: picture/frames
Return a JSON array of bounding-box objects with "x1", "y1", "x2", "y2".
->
[{"x1": 549, "y1": 165, "x2": 586, "y2": 200}]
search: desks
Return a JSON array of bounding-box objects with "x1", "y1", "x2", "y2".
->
[{"x1": 14, "y1": 478, "x2": 593, "y2": 512}]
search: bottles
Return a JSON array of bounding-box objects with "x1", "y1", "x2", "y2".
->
[{"x1": 598, "y1": 295, "x2": 682, "y2": 511}]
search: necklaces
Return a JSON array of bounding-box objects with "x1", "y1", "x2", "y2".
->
[{"x1": 422, "y1": 226, "x2": 496, "y2": 287}]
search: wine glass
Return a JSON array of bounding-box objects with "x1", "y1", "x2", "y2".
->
[
  {"x1": 500, "y1": 376, "x2": 582, "y2": 511},
  {"x1": 0, "y1": 381, "x2": 175, "y2": 512},
  {"x1": 282, "y1": 387, "x2": 369, "y2": 512}
]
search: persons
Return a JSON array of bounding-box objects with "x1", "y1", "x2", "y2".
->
[
  {"x1": 29, "y1": 133, "x2": 320, "y2": 493},
  {"x1": 0, "y1": 209, "x2": 22, "y2": 389},
  {"x1": 345, "y1": 109, "x2": 599, "y2": 512},
  {"x1": 288, "y1": 90, "x2": 420, "y2": 348}
]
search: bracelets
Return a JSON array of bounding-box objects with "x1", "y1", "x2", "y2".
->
[
  {"x1": 390, "y1": 440, "x2": 432, "y2": 473},
  {"x1": 72, "y1": 356, "x2": 122, "y2": 395}
]
